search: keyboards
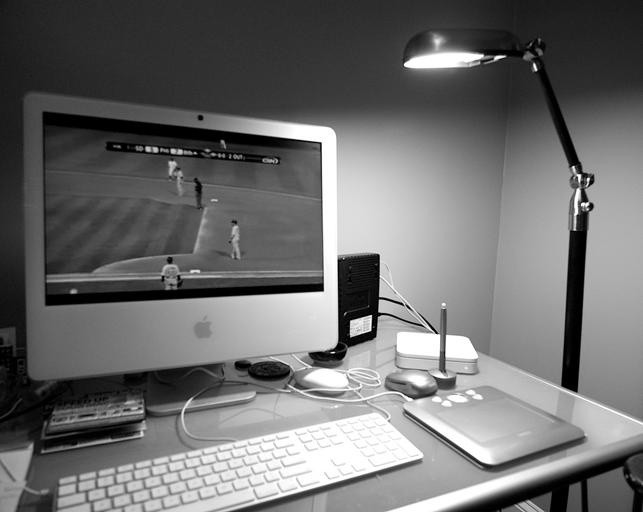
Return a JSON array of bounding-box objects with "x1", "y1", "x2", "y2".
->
[{"x1": 50, "y1": 413, "x2": 423, "y2": 512}]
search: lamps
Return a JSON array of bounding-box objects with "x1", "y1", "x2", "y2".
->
[{"x1": 401, "y1": 29, "x2": 594, "y2": 511}]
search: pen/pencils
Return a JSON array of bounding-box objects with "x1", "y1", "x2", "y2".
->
[{"x1": 438, "y1": 303, "x2": 447, "y2": 371}]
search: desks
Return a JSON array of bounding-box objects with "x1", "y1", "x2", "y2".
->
[{"x1": 0, "y1": 320, "x2": 643, "y2": 512}]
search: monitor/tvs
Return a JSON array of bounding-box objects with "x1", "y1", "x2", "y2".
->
[{"x1": 25, "y1": 92, "x2": 339, "y2": 418}]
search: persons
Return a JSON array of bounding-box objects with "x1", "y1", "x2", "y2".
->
[
  {"x1": 225, "y1": 216, "x2": 241, "y2": 263},
  {"x1": 193, "y1": 176, "x2": 202, "y2": 210},
  {"x1": 160, "y1": 255, "x2": 182, "y2": 291},
  {"x1": 174, "y1": 166, "x2": 184, "y2": 197},
  {"x1": 167, "y1": 157, "x2": 178, "y2": 180}
]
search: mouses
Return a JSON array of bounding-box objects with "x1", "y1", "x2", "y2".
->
[
  {"x1": 385, "y1": 369, "x2": 441, "y2": 398},
  {"x1": 293, "y1": 366, "x2": 350, "y2": 397}
]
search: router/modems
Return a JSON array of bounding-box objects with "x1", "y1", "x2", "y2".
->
[{"x1": 396, "y1": 331, "x2": 478, "y2": 375}]
